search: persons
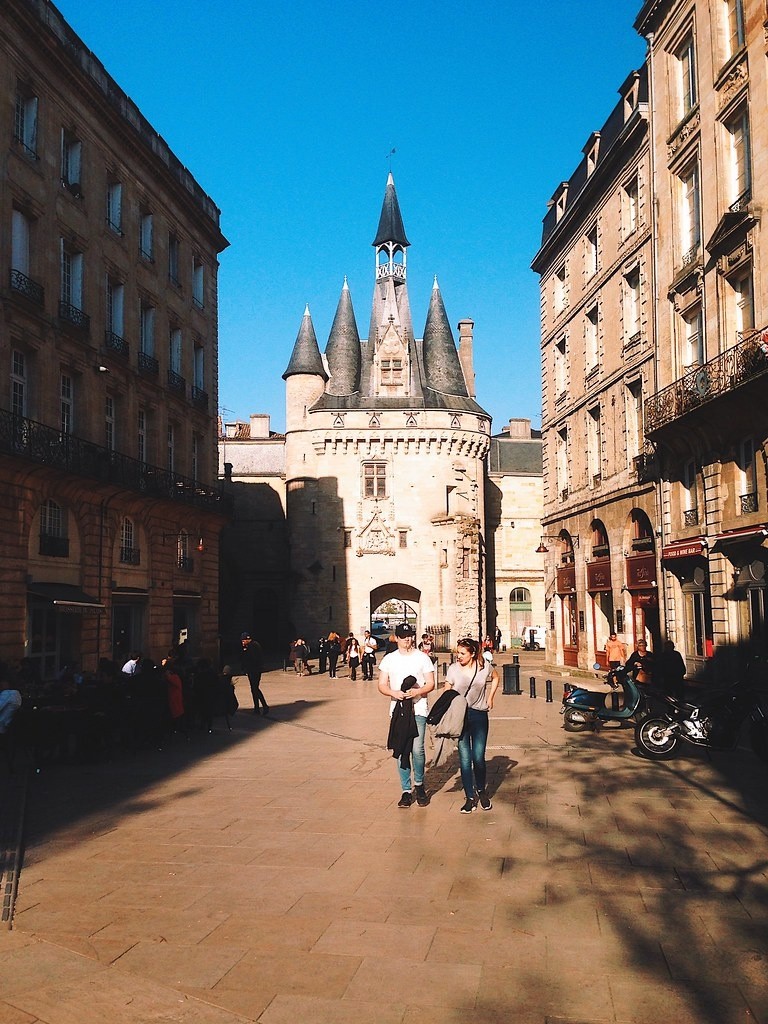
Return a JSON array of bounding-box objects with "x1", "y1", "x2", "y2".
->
[
  {"x1": 606, "y1": 632, "x2": 627, "y2": 690},
  {"x1": 290, "y1": 625, "x2": 502, "y2": 681},
  {"x1": 444, "y1": 639, "x2": 499, "y2": 813},
  {"x1": 626, "y1": 639, "x2": 656, "y2": 683},
  {"x1": 0, "y1": 636, "x2": 239, "y2": 734},
  {"x1": 241, "y1": 632, "x2": 269, "y2": 714},
  {"x1": 378, "y1": 624, "x2": 434, "y2": 808},
  {"x1": 662, "y1": 641, "x2": 686, "y2": 679}
]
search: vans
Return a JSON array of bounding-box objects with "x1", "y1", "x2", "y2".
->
[{"x1": 520, "y1": 626, "x2": 547, "y2": 651}]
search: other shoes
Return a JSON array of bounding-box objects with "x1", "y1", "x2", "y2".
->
[
  {"x1": 253, "y1": 709, "x2": 259, "y2": 713},
  {"x1": 363, "y1": 675, "x2": 368, "y2": 680},
  {"x1": 263, "y1": 706, "x2": 269, "y2": 715},
  {"x1": 369, "y1": 675, "x2": 372, "y2": 680},
  {"x1": 351, "y1": 675, "x2": 355, "y2": 680}
]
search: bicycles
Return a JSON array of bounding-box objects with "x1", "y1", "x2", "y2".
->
[{"x1": 296, "y1": 660, "x2": 303, "y2": 677}]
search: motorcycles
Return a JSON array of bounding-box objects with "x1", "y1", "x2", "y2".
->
[{"x1": 635, "y1": 655, "x2": 768, "y2": 765}]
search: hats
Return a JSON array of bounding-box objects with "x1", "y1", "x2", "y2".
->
[
  {"x1": 240, "y1": 632, "x2": 252, "y2": 639},
  {"x1": 395, "y1": 624, "x2": 414, "y2": 639}
]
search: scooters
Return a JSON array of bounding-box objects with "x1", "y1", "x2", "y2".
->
[{"x1": 559, "y1": 663, "x2": 652, "y2": 732}]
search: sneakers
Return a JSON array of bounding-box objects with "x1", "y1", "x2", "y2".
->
[
  {"x1": 398, "y1": 792, "x2": 413, "y2": 807},
  {"x1": 414, "y1": 784, "x2": 428, "y2": 806},
  {"x1": 460, "y1": 796, "x2": 477, "y2": 814},
  {"x1": 476, "y1": 789, "x2": 491, "y2": 810}
]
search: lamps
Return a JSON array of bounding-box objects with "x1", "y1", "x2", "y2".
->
[
  {"x1": 536, "y1": 534, "x2": 579, "y2": 553},
  {"x1": 163, "y1": 531, "x2": 207, "y2": 551}
]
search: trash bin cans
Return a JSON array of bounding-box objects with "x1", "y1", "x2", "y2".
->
[{"x1": 502, "y1": 664, "x2": 524, "y2": 695}]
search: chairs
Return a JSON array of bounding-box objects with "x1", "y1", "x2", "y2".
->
[{"x1": 10, "y1": 673, "x2": 233, "y2": 774}]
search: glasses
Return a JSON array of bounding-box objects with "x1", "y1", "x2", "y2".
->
[{"x1": 457, "y1": 640, "x2": 476, "y2": 652}]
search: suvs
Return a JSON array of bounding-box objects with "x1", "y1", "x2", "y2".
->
[{"x1": 372, "y1": 629, "x2": 390, "y2": 649}]
